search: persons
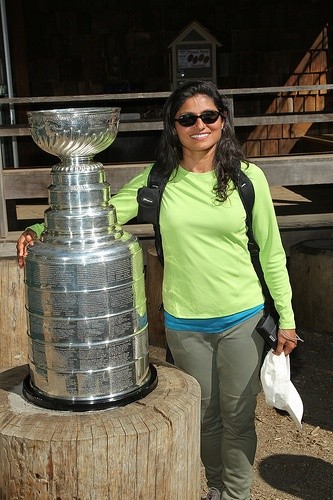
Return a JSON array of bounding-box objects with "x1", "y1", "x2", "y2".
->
[{"x1": 16, "y1": 79, "x2": 299, "y2": 500}]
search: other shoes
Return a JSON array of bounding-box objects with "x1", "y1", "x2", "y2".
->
[{"x1": 205, "y1": 486, "x2": 221, "y2": 500}]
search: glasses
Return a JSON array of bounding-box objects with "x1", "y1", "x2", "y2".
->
[{"x1": 174, "y1": 110, "x2": 225, "y2": 127}]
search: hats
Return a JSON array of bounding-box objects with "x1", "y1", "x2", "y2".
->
[{"x1": 260, "y1": 348, "x2": 304, "y2": 427}]
